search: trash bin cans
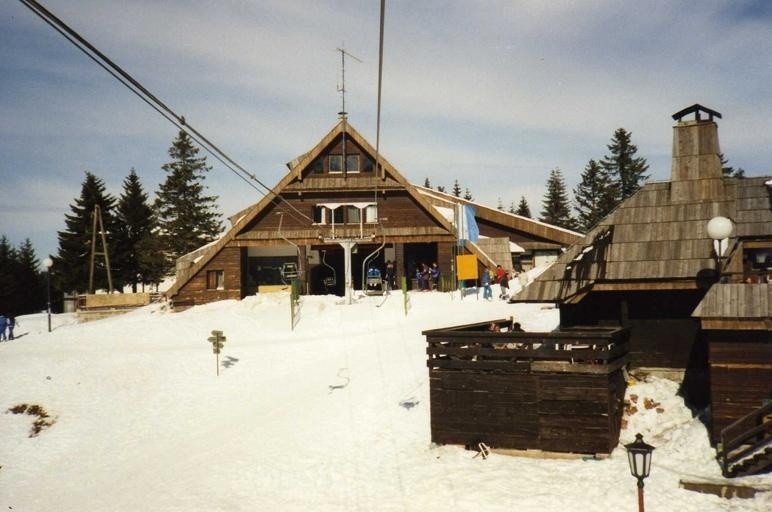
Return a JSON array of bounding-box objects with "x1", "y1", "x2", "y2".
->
[
  {"x1": 64, "y1": 295, "x2": 75, "y2": 313},
  {"x1": 411, "y1": 279, "x2": 418, "y2": 290}
]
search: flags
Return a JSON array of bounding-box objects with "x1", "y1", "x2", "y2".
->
[{"x1": 456, "y1": 202, "x2": 480, "y2": 248}]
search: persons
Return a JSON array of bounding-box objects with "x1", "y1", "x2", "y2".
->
[
  {"x1": 6, "y1": 314, "x2": 20, "y2": 340},
  {"x1": 518, "y1": 269, "x2": 528, "y2": 287},
  {"x1": 494, "y1": 264, "x2": 505, "y2": 289},
  {"x1": 0, "y1": 313, "x2": 7, "y2": 342},
  {"x1": 386, "y1": 260, "x2": 394, "y2": 288},
  {"x1": 415, "y1": 261, "x2": 440, "y2": 291},
  {"x1": 481, "y1": 265, "x2": 493, "y2": 301},
  {"x1": 498, "y1": 272, "x2": 510, "y2": 300}
]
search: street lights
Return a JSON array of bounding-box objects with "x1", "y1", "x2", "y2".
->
[
  {"x1": 44, "y1": 258, "x2": 53, "y2": 332},
  {"x1": 624, "y1": 433, "x2": 656, "y2": 512}
]
